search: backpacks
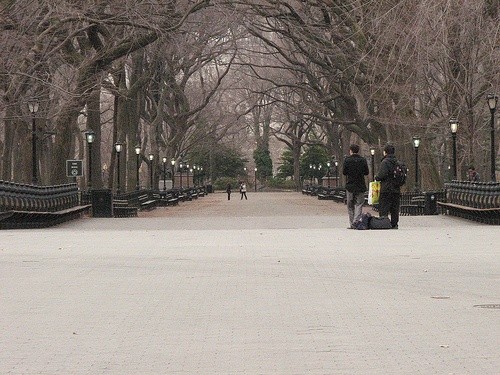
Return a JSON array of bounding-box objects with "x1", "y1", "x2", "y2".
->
[{"x1": 385, "y1": 158, "x2": 407, "y2": 188}]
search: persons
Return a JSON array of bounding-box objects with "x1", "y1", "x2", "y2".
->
[
  {"x1": 343, "y1": 145, "x2": 369, "y2": 228},
  {"x1": 239, "y1": 182, "x2": 247, "y2": 200},
  {"x1": 376, "y1": 145, "x2": 400, "y2": 228},
  {"x1": 227, "y1": 184, "x2": 233, "y2": 200}
]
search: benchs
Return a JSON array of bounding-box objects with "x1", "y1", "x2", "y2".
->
[
  {"x1": 435, "y1": 180, "x2": 500, "y2": 225},
  {"x1": 153, "y1": 188, "x2": 204, "y2": 206},
  {"x1": 303, "y1": 186, "x2": 347, "y2": 204},
  {"x1": 399, "y1": 196, "x2": 425, "y2": 216},
  {"x1": 137, "y1": 194, "x2": 156, "y2": 212},
  {"x1": 113, "y1": 200, "x2": 138, "y2": 217},
  {"x1": 0, "y1": 180, "x2": 93, "y2": 230}
]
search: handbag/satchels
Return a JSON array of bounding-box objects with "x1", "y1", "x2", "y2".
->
[
  {"x1": 367, "y1": 214, "x2": 392, "y2": 229},
  {"x1": 368, "y1": 180, "x2": 381, "y2": 205},
  {"x1": 352, "y1": 212, "x2": 371, "y2": 230}
]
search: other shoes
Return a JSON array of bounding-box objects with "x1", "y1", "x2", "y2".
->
[{"x1": 393, "y1": 225, "x2": 398, "y2": 228}]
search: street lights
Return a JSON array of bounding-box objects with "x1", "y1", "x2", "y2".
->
[
  {"x1": 179, "y1": 161, "x2": 184, "y2": 197},
  {"x1": 326, "y1": 160, "x2": 331, "y2": 188},
  {"x1": 369, "y1": 145, "x2": 376, "y2": 182},
  {"x1": 85, "y1": 129, "x2": 96, "y2": 196},
  {"x1": 334, "y1": 159, "x2": 340, "y2": 189},
  {"x1": 193, "y1": 164, "x2": 204, "y2": 193},
  {"x1": 27, "y1": 95, "x2": 40, "y2": 186},
  {"x1": 412, "y1": 133, "x2": 422, "y2": 194},
  {"x1": 135, "y1": 142, "x2": 141, "y2": 197},
  {"x1": 486, "y1": 93, "x2": 499, "y2": 182},
  {"x1": 170, "y1": 158, "x2": 177, "y2": 195},
  {"x1": 308, "y1": 163, "x2": 322, "y2": 187},
  {"x1": 185, "y1": 161, "x2": 190, "y2": 191},
  {"x1": 148, "y1": 151, "x2": 155, "y2": 194},
  {"x1": 448, "y1": 114, "x2": 460, "y2": 179},
  {"x1": 162, "y1": 155, "x2": 168, "y2": 194},
  {"x1": 114, "y1": 139, "x2": 123, "y2": 198},
  {"x1": 254, "y1": 167, "x2": 258, "y2": 193}
]
